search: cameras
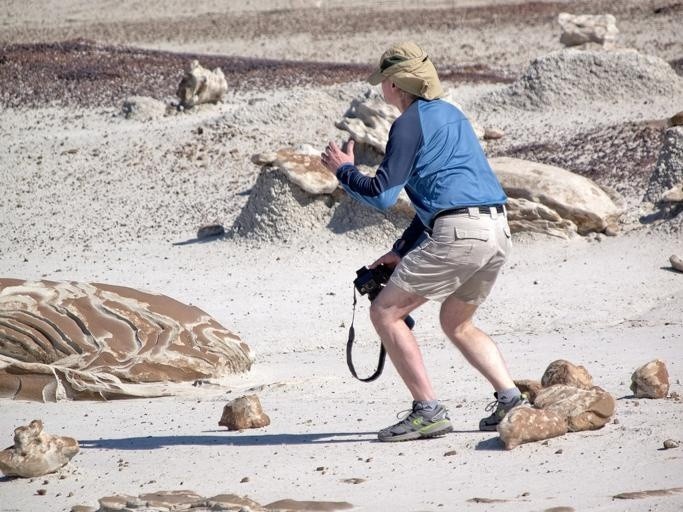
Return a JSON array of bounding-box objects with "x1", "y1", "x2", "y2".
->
[{"x1": 352, "y1": 262, "x2": 415, "y2": 330}]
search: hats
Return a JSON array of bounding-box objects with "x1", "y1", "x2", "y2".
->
[{"x1": 365, "y1": 39, "x2": 445, "y2": 104}]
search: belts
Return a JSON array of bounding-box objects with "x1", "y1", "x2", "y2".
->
[{"x1": 427, "y1": 204, "x2": 505, "y2": 231}]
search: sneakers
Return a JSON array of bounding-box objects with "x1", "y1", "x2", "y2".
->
[
  {"x1": 477, "y1": 392, "x2": 533, "y2": 432},
  {"x1": 377, "y1": 399, "x2": 453, "y2": 444}
]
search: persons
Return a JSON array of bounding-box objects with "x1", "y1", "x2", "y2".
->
[{"x1": 321, "y1": 40, "x2": 532, "y2": 442}]
